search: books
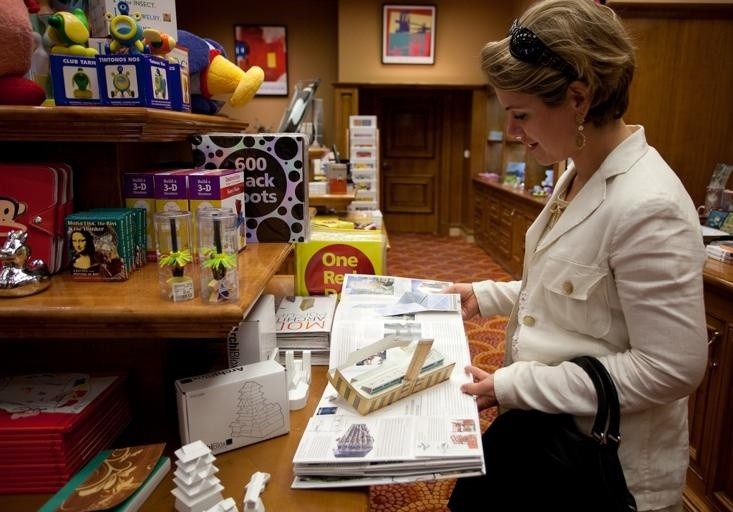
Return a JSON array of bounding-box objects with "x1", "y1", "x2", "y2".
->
[
  {"x1": 292, "y1": 271, "x2": 487, "y2": 488},
  {"x1": 64, "y1": 204, "x2": 148, "y2": 282}
]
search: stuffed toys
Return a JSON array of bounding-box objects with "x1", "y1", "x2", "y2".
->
[{"x1": 1, "y1": 0, "x2": 265, "y2": 125}]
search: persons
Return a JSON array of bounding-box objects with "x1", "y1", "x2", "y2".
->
[{"x1": 440, "y1": 1, "x2": 711, "y2": 512}]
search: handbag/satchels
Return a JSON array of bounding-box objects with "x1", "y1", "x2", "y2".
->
[{"x1": 446, "y1": 358, "x2": 637, "y2": 510}]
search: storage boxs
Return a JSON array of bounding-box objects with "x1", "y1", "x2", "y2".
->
[{"x1": 49, "y1": 0, "x2": 191, "y2": 111}]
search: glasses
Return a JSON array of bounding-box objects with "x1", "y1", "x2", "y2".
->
[{"x1": 505, "y1": 19, "x2": 582, "y2": 82}]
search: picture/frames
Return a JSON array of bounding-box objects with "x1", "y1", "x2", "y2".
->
[
  {"x1": 232, "y1": 21, "x2": 289, "y2": 98},
  {"x1": 381, "y1": 3, "x2": 437, "y2": 65}
]
